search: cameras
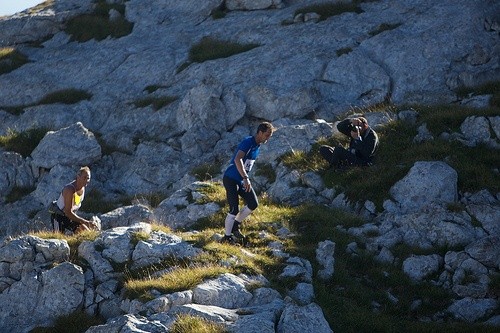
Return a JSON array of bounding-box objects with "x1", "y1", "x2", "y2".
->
[{"x1": 351, "y1": 123, "x2": 362, "y2": 133}]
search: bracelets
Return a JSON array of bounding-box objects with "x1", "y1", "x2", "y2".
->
[{"x1": 242, "y1": 175, "x2": 249, "y2": 179}]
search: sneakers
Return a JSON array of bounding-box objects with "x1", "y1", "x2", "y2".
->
[
  {"x1": 221, "y1": 235, "x2": 236, "y2": 245},
  {"x1": 232, "y1": 220, "x2": 246, "y2": 238}
]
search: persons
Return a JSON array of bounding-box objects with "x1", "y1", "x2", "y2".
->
[
  {"x1": 51, "y1": 166, "x2": 98, "y2": 236},
  {"x1": 223, "y1": 122, "x2": 273, "y2": 245},
  {"x1": 320, "y1": 117, "x2": 379, "y2": 173}
]
